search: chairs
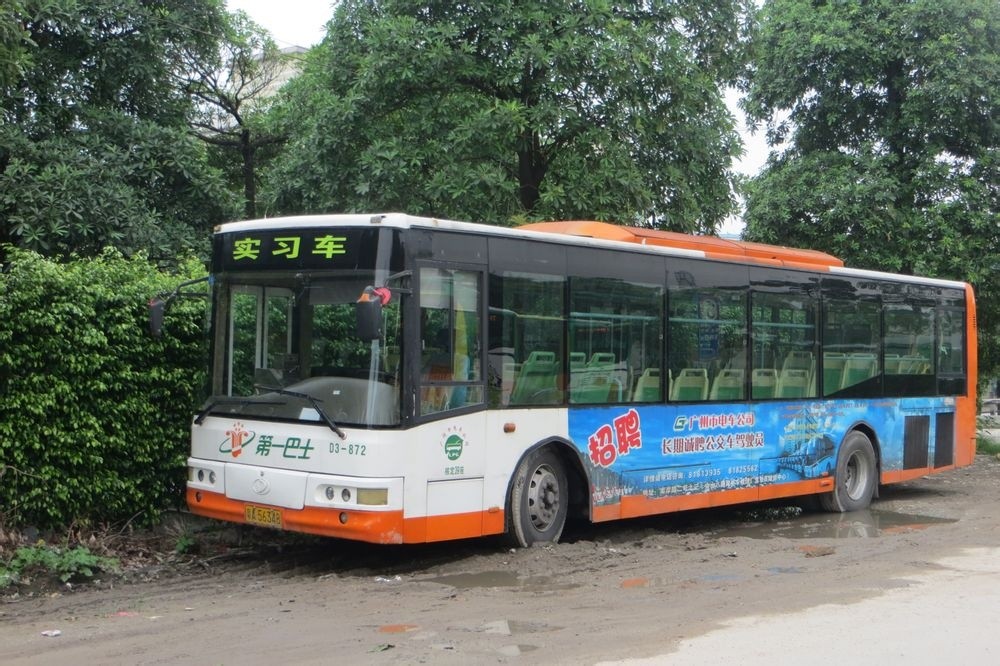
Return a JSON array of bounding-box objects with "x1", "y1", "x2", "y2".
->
[{"x1": 382, "y1": 345, "x2": 931, "y2": 415}]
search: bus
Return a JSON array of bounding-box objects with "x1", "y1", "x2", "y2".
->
[{"x1": 150, "y1": 210, "x2": 980, "y2": 551}]
